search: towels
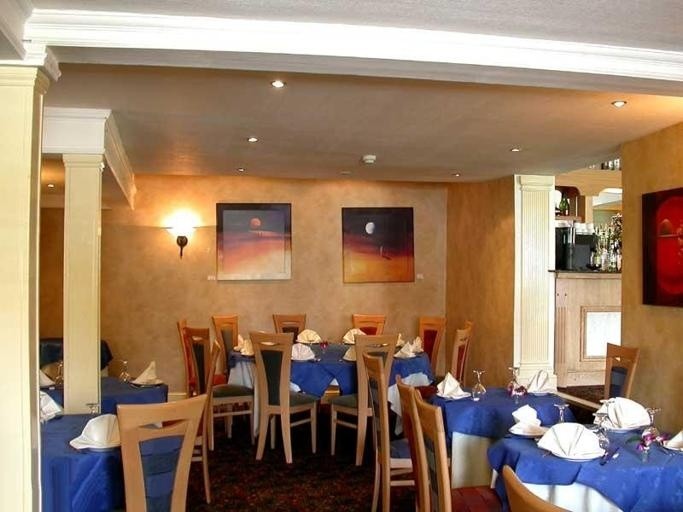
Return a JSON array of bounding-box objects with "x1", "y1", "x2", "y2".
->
[
  {"x1": 412, "y1": 336, "x2": 423, "y2": 353},
  {"x1": 69, "y1": 413, "x2": 122, "y2": 450},
  {"x1": 38, "y1": 368, "x2": 55, "y2": 389},
  {"x1": 394, "y1": 341, "x2": 416, "y2": 358},
  {"x1": 295, "y1": 328, "x2": 321, "y2": 344},
  {"x1": 436, "y1": 370, "x2": 471, "y2": 399},
  {"x1": 342, "y1": 328, "x2": 366, "y2": 344},
  {"x1": 234, "y1": 333, "x2": 244, "y2": 351},
  {"x1": 593, "y1": 396, "x2": 651, "y2": 429},
  {"x1": 291, "y1": 343, "x2": 315, "y2": 360},
  {"x1": 241, "y1": 339, "x2": 254, "y2": 356},
  {"x1": 526, "y1": 369, "x2": 558, "y2": 393},
  {"x1": 536, "y1": 422, "x2": 603, "y2": 459},
  {"x1": 508, "y1": 403, "x2": 541, "y2": 435},
  {"x1": 132, "y1": 361, "x2": 157, "y2": 385},
  {"x1": 39, "y1": 391, "x2": 62, "y2": 422},
  {"x1": 343, "y1": 346, "x2": 358, "y2": 360}
]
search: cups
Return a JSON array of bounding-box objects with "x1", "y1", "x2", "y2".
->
[
  {"x1": 54, "y1": 361, "x2": 65, "y2": 388},
  {"x1": 506, "y1": 366, "x2": 521, "y2": 396},
  {"x1": 590, "y1": 413, "x2": 610, "y2": 450},
  {"x1": 471, "y1": 369, "x2": 487, "y2": 400},
  {"x1": 600, "y1": 398, "x2": 614, "y2": 438},
  {"x1": 640, "y1": 407, "x2": 663, "y2": 448},
  {"x1": 118, "y1": 359, "x2": 130, "y2": 383}
]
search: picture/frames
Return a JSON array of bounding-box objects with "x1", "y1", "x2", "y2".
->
[
  {"x1": 215, "y1": 202, "x2": 294, "y2": 281},
  {"x1": 342, "y1": 206, "x2": 415, "y2": 284},
  {"x1": 641, "y1": 187, "x2": 682, "y2": 309}
]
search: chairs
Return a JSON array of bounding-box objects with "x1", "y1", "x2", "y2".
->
[
  {"x1": 328, "y1": 333, "x2": 399, "y2": 466},
  {"x1": 191, "y1": 333, "x2": 256, "y2": 451},
  {"x1": 416, "y1": 315, "x2": 447, "y2": 379},
  {"x1": 273, "y1": 313, "x2": 308, "y2": 343},
  {"x1": 248, "y1": 330, "x2": 320, "y2": 464},
  {"x1": 418, "y1": 316, "x2": 447, "y2": 386},
  {"x1": 414, "y1": 320, "x2": 471, "y2": 399},
  {"x1": 211, "y1": 313, "x2": 241, "y2": 375},
  {"x1": 394, "y1": 372, "x2": 453, "y2": 512},
  {"x1": 500, "y1": 463, "x2": 570, "y2": 512},
  {"x1": 414, "y1": 388, "x2": 502, "y2": 511},
  {"x1": 352, "y1": 313, "x2": 386, "y2": 338},
  {"x1": 175, "y1": 318, "x2": 228, "y2": 396},
  {"x1": 487, "y1": 422, "x2": 683, "y2": 512},
  {"x1": 599, "y1": 342, "x2": 640, "y2": 400},
  {"x1": 114, "y1": 394, "x2": 208, "y2": 511},
  {"x1": 361, "y1": 345, "x2": 424, "y2": 511},
  {"x1": 163, "y1": 340, "x2": 222, "y2": 452}
]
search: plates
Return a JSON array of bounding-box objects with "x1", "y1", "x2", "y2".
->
[
  {"x1": 562, "y1": 456, "x2": 597, "y2": 463},
  {"x1": 394, "y1": 354, "x2": 418, "y2": 359},
  {"x1": 530, "y1": 392, "x2": 550, "y2": 397},
  {"x1": 659, "y1": 440, "x2": 683, "y2": 453},
  {"x1": 342, "y1": 356, "x2": 358, "y2": 362},
  {"x1": 133, "y1": 379, "x2": 164, "y2": 386},
  {"x1": 611, "y1": 428, "x2": 634, "y2": 433},
  {"x1": 240, "y1": 353, "x2": 255, "y2": 356},
  {"x1": 509, "y1": 428, "x2": 550, "y2": 438},
  {"x1": 88, "y1": 447, "x2": 119, "y2": 452},
  {"x1": 436, "y1": 393, "x2": 468, "y2": 401},
  {"x1": 291, "y1": 358, "x2": 315, "y2": 363}
]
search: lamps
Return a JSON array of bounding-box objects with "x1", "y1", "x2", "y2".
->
[{"x1": 163, "y1": 208, "x2": 203, "y2": 259}]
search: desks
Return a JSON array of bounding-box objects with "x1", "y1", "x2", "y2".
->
[
  {"x1": 229, "y1": 338, "x2": 434, "y2": 438},
  {"x1": 39, "y1": 337, "x2": 110, "y2": 383},
  {"x1": 40, "y1": 375, "x2": 169, "y2": 416},
  {"x1": 41, "y1": 414, "x2": 187, "y2": 512},
  {"x1": 427, "y1": 383, "x2": 572, "y2": 489}
]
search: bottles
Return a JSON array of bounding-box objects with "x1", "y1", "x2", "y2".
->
[{"x1": 559, "y1": 187, "x2": 569, "y2": 216}]
